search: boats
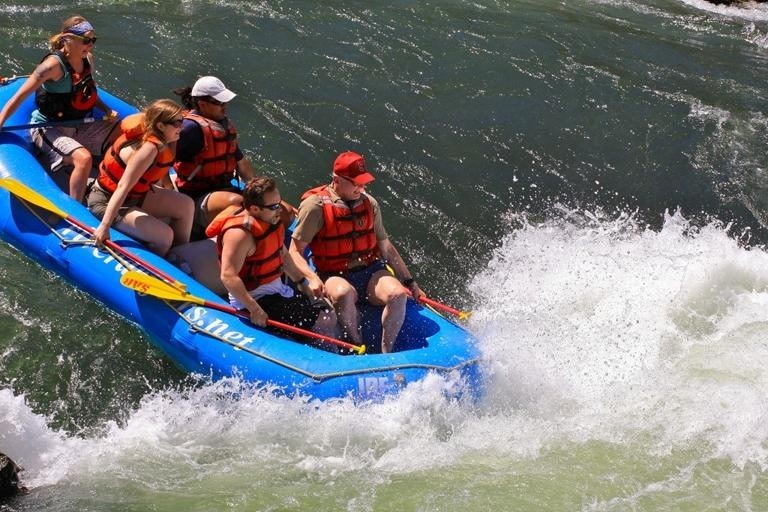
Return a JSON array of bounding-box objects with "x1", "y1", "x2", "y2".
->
[{"x1": 0, "y1": 74, "x2": 489, "y2": 403}]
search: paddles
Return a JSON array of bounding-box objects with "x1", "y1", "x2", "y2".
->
[
  {"x1": 0, "y1": 176, "x2": 187, "y2": 289},
  {"x1": 119, "y1": 271, "x2": 367, "y2": 356},
  {"x1": 386, "y1": 264, "x2": 471, "y2": 321}
]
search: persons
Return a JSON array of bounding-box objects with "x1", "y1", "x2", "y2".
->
[
  {"x1": 206, "y1": 176, "x2": 341, "y2": 356},
  {"x1": 172, "y1": 75, "x2": 296, "y2": 235},
  {"x1": 0, "y1": 16, "x2": 122, "y2": 205},
  {"x1": 87, "y1": 99, "x2": 196, "y2": 260},
  {"x1": 287, "y1": 152, "x2": 427, "y2": 354}
]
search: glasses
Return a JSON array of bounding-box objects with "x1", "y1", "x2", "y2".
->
[
  {"x1": 82, "y1": 36, "x2": 97, "y2": 45},
  {"x1": 261, "y1": 203, "x2": 281, "y2": 212},
  {"x1": 165, "y1": 115, "x2": 183, "y2": 127}
]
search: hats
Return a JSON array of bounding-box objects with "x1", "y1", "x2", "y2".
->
[
  {"x1": 334, "y1": 152, "x2": 376, "y2": 186},
  {"x1": 190, "y1": 77, "x2": 238, "y2": 105}
]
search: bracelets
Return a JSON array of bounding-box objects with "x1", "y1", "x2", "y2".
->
[
  {"x1": 404, "y1": 275, "x2": 416, "y2": 287},
  {"x1": 293, "y1": 276, "x2": 306, "y2": 286}
]
